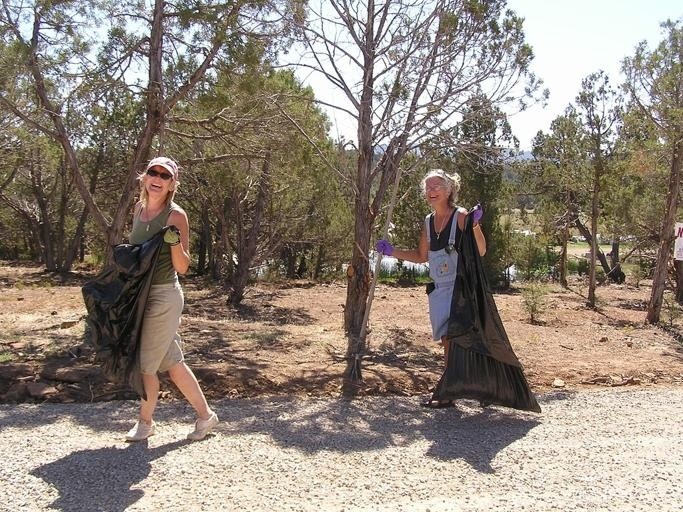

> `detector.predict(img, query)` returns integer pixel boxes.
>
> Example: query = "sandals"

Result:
[420,398,493,409]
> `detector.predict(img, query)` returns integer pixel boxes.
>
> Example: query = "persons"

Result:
[373,169,492,408]
[126,157,220,442]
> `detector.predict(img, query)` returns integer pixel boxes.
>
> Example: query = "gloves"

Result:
[471,203,484,223]
[375,239,394,257]
[163,224,182,246]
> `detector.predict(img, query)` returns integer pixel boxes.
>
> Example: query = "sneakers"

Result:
[124,420,157,440]
[187,413,219,440]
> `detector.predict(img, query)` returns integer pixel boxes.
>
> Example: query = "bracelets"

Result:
[472,222,479,230]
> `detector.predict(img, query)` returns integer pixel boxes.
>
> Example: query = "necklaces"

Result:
[434,205,450,240]
[146,200,167,232]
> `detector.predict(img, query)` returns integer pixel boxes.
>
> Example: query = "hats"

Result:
[146,156,179,176]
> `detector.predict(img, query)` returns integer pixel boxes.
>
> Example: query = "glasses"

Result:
[147,169,173,180]
[424,184,449,191]
